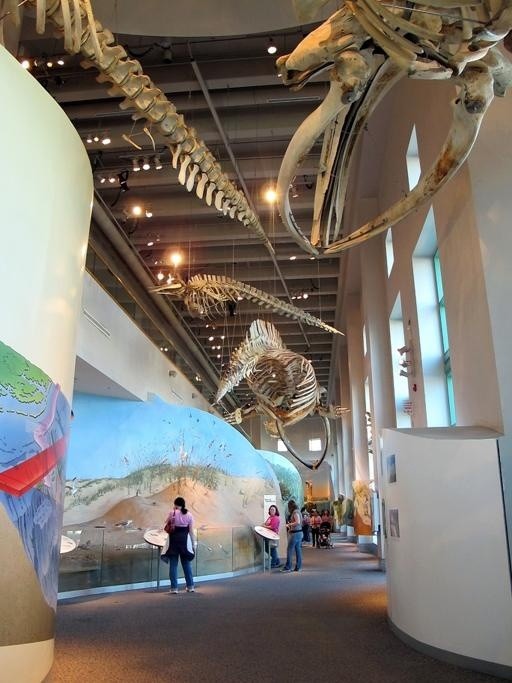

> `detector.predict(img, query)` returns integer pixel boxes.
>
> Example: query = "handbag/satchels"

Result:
[164,518,175,532]
[269,540,279,548]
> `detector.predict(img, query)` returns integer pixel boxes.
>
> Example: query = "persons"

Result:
[262,504,284,568]
[278,499,305,572]
[164,497,197,594]
[300,504,333,550]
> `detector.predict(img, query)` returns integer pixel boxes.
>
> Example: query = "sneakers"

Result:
[185,586,194,593]
[268,562,301,572]
[170,588,178,594]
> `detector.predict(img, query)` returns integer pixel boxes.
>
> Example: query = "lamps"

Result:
[81,134,189,291]
[398,338,416,379]
[204,322,225,368]
[290,287,310,300]
[20,53,67,72]
[262,180,299,203]
[266,36,278,54]
